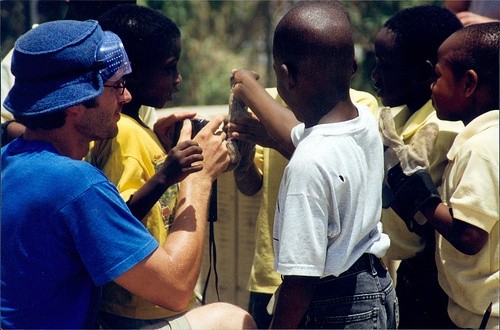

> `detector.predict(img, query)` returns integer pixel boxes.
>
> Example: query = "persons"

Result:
[0,0,500,330]
[0,19,259,330]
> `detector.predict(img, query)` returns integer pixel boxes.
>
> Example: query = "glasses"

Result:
[104,81,126,96]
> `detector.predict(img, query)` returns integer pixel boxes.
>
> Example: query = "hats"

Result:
[2,19,131,116]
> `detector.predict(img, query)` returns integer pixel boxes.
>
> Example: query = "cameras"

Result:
[172,119,209,146]
